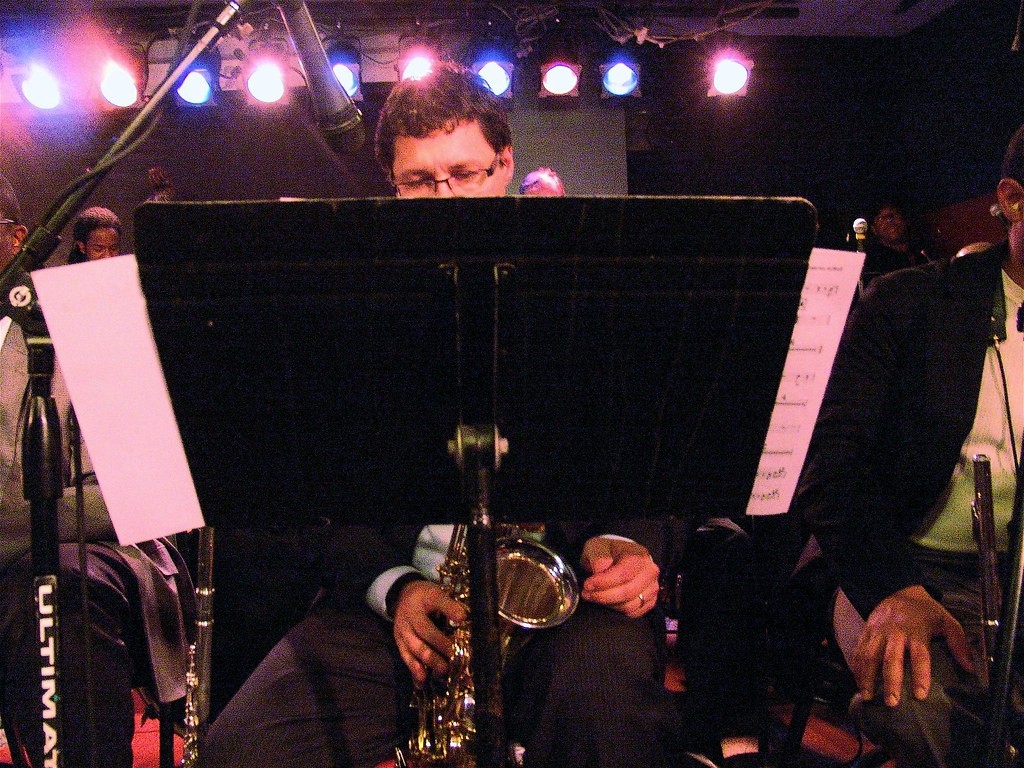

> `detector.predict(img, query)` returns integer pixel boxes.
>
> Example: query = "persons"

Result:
[866,201,928,281]
[194,62,678,768]
[808,120,1024,767]
[68,206,123,266]
[0,170,197,768]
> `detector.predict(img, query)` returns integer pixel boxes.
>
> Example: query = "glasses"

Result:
[386,151,499,200]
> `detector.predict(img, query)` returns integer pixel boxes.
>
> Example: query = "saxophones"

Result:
[392,519,579,768]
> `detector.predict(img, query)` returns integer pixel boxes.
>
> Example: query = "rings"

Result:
[639,594,645,605]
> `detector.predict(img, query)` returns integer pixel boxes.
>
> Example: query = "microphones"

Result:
[853,218,869,252]
[274,0,366,152]
[991,203,1011,233]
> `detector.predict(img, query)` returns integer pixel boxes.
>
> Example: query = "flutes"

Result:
[972,452,1001,689]
[182,527,215,768]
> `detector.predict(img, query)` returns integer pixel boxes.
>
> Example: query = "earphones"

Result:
[1013,202,1022,213]
